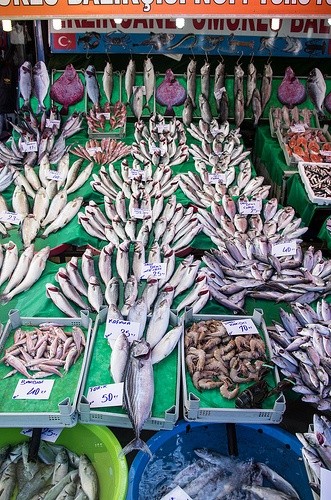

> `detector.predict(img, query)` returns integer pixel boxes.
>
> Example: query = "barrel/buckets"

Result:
[126,419,317,500]
[0,418,129,499]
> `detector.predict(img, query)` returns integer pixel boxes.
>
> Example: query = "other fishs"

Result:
[0,57,330,500]
